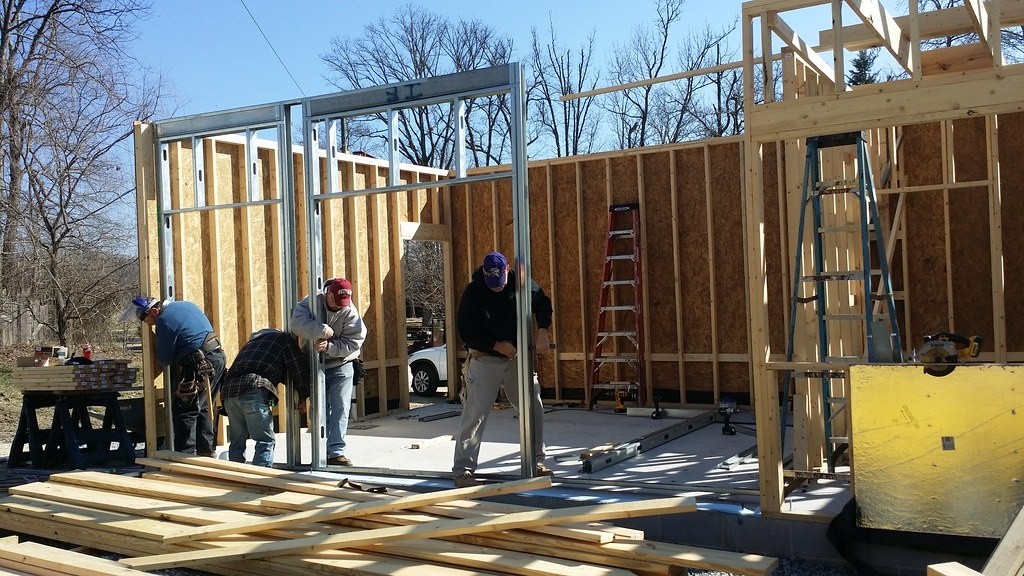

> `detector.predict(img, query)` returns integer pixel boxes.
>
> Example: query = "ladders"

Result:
[780,130,904,499]
[587,203,646,411]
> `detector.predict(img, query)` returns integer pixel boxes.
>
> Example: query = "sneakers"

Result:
[454,470,479,488]
[534,463,553,475]
[327,455,352,466]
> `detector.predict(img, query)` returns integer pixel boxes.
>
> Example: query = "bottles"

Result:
[58,346,66,366]
[82,341,92,359]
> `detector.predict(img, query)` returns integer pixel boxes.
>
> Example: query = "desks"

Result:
[7,390,135,470]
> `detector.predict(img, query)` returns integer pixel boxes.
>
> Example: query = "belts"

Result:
[207,347,222,355]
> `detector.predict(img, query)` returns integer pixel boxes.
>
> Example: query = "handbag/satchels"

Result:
[353,358,368,386]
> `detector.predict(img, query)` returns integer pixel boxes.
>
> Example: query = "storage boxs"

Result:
[34,345,67,367]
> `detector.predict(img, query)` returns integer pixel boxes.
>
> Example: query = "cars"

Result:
[408,342,449,397]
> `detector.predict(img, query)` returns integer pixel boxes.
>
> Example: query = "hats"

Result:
[482,252,508,288]
[330,277,353,306]
[134,296,159,321]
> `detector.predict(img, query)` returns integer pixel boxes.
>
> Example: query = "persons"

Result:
[451,252,554,488]
[132,298,226,458]
[221,329,310,469]
[290,278,368,466]
